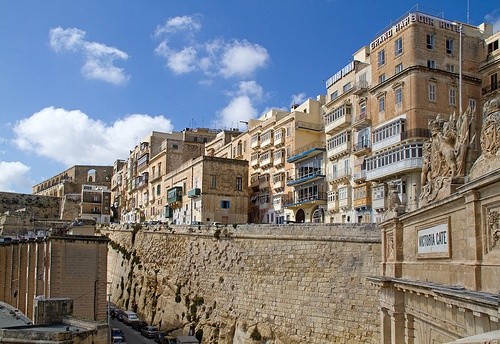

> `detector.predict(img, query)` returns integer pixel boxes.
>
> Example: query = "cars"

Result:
[125,221,225,227]
[163,335,175,344]
[141,326,160,339]
[109,304,145,344]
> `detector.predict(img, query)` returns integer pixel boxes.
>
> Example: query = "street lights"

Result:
[451,21,464,117]
